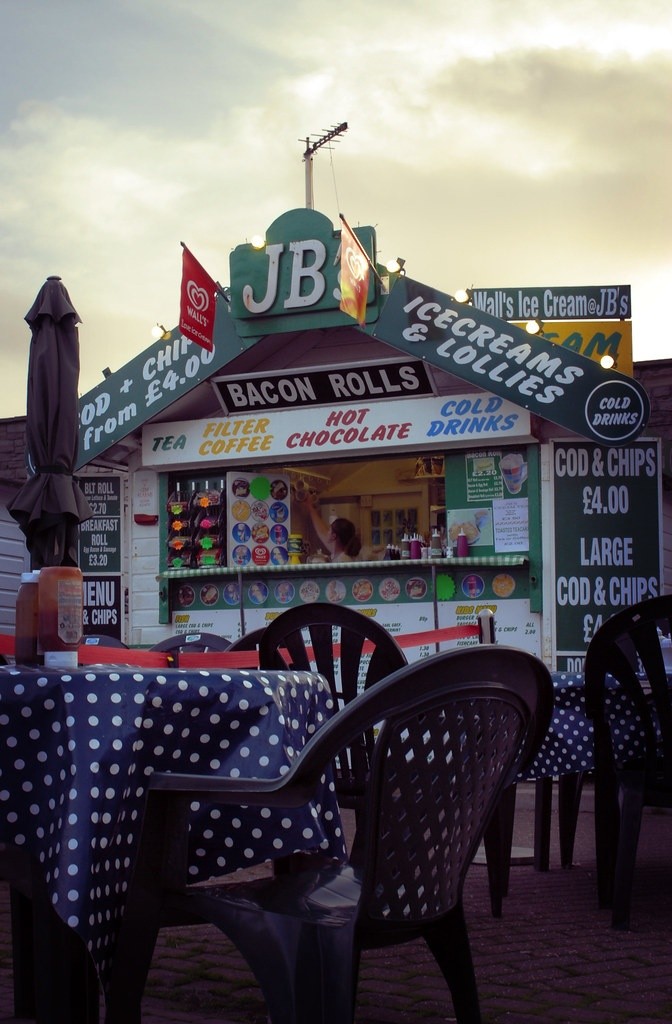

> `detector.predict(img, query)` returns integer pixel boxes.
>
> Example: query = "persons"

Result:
[304,496,361,563]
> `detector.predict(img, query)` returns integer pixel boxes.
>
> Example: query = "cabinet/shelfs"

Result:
[414,474,445,514]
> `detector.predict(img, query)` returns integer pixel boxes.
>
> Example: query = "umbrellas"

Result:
[6,277,94,567]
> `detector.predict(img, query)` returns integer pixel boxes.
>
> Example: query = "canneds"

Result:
[288,534,303,564]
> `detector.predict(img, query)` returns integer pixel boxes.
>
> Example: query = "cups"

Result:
[502,462,529,494]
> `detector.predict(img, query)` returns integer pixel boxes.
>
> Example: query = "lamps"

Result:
[387,257,406,276]
[526,317,545,336]
[454,287,474,305]
[600,352,619,369]
[151,322,167,340]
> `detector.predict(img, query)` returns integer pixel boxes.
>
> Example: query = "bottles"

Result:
[15,572,44,666]
[38,566,83,666]
[383,533,427,560]
[468,576,476,597]
[457,528,469,557]
[431,528,442,558]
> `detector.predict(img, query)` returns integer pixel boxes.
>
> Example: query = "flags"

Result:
[180,243,220,353]
[336,213,371,330]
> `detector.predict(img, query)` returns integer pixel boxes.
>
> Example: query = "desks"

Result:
[0,665,350,1024]
[528,671,672,871]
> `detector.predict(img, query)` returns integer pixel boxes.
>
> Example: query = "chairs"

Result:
[79,629,269,652]
[584,594,672,930]
[106,644,556,1024]
[259,602,515,918]
